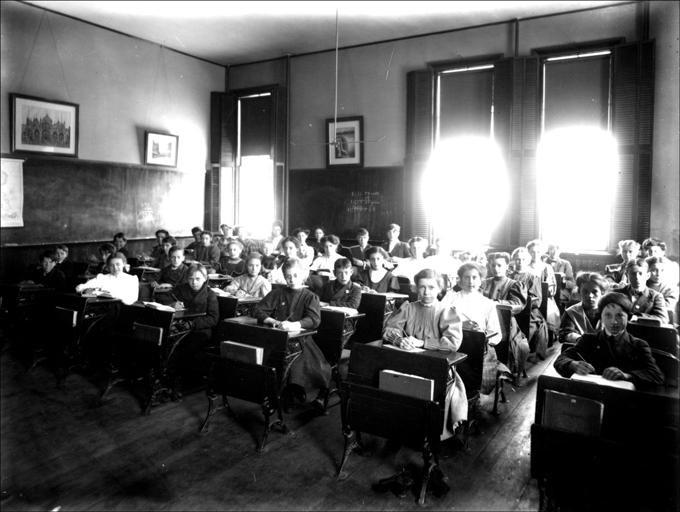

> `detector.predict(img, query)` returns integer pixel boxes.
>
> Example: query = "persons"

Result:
[380,239,575,396]
[556,237,680,389]
[24,223,463,334]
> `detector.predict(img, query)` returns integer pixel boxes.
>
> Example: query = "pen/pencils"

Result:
[402,330,410,340]
[576,351,596,374]
[357,280,365,286]
[170,292,179,301]
[463,313,471,321]
[234,282,241,290]
[284,312,294,321]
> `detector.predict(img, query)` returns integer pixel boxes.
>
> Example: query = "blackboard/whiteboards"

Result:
[288,165,406,243]
[0,152,205,248]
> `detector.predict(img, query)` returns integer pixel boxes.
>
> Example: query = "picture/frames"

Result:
[323,115,364,167]
[144,129,177,170]
[7,90,79,156]
